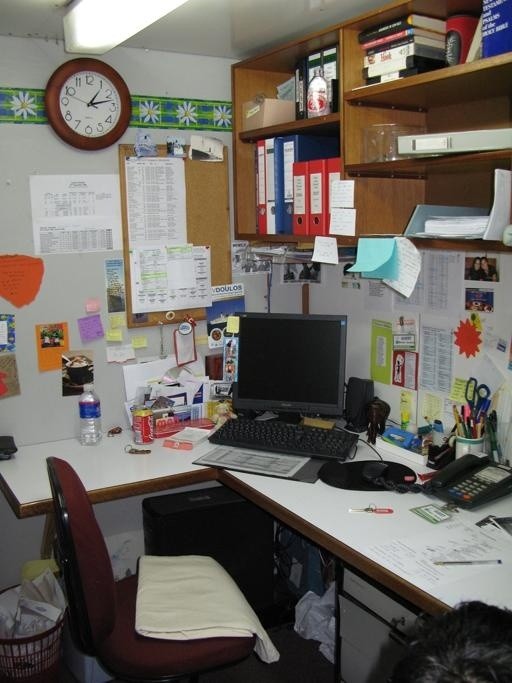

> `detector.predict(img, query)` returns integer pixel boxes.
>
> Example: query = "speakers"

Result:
[232,381,257,418]
[344,377,374,434]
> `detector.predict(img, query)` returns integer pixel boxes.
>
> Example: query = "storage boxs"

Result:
[238,94,294,134]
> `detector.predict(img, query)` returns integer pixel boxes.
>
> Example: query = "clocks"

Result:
[43,56,133,151]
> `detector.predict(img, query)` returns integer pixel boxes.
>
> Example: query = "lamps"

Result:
[61,0,187,54]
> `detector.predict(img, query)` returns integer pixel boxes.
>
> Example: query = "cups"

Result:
[455,434,485,461]
[64,359,93,384]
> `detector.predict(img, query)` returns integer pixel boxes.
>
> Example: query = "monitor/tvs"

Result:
[237,311,347,425]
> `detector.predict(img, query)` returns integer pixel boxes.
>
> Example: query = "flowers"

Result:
[174,101,198,127]
[9,91,38,119]
[137,100,161,124]
[213,104,232,128]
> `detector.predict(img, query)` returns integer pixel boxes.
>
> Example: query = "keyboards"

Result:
[208,418,360,461]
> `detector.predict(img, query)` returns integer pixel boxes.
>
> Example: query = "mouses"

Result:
[361,462,388,481]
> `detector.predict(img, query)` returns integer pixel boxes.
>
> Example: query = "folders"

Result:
[258,135,341,236]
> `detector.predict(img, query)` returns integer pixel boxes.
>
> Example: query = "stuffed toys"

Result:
[207,402,238,437]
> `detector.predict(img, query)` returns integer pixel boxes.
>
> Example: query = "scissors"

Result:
[465,377,490,418]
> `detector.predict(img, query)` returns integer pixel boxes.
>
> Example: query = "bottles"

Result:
[78,383,101,444]
[306,68,330,119]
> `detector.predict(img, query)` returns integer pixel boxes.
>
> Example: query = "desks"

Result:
[0,406,512,682]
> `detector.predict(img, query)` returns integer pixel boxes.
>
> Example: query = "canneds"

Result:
[133,406,153,445]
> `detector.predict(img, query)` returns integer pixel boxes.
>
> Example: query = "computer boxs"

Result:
[142,486,274,631]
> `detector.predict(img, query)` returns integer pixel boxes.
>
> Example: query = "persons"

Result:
[468,257,483,280]
[225,339,234,358]
[225,360,233,383]
[480,257,497,282]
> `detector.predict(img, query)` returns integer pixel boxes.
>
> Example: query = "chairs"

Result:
[41,454,259,682]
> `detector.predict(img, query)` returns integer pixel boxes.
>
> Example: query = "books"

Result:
[350,0,512,92]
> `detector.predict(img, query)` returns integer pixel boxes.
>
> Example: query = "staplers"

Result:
[427,445,455,470]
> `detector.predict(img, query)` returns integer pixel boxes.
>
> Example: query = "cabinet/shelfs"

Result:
[345,0,512,254]
[227,25,351,249]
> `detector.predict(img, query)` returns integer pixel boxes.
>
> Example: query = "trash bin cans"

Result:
[0,584,65,683]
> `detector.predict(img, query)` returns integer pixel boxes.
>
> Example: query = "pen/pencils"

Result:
[453,405,500,464]
[434,561,502,565]
